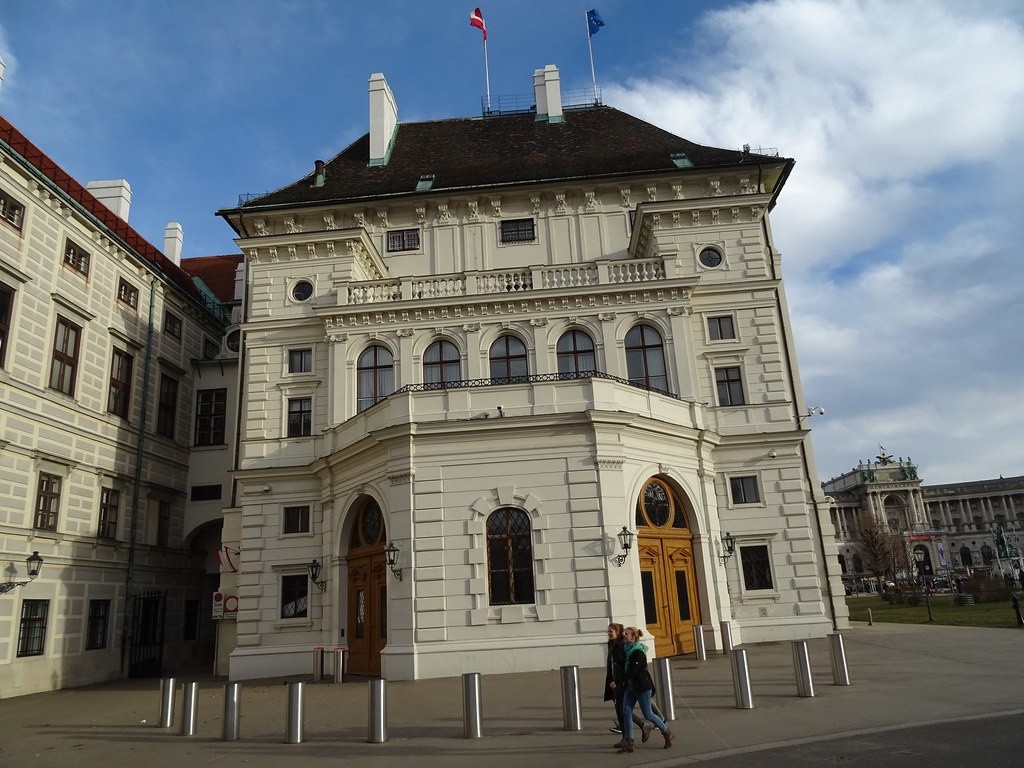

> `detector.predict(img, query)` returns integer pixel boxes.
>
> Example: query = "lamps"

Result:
[0,551,44,594]
[384,542,402,582]
[308,558,327,593]
[719,532,737,566]
[618,525,634,567]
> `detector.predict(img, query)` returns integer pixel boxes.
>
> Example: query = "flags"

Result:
[469,7,487,42]
[586,9,606,39]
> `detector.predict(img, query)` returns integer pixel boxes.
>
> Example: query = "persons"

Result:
[603,624,667,749]
[616,627,674,754]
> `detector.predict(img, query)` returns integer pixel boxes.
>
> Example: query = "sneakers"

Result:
[609,725,622,734]
[653,718,668,730]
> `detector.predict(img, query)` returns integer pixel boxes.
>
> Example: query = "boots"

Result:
[617,742,634,754]
[661,728,676,749]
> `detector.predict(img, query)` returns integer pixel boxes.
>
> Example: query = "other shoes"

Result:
[641,723,652,743]
[614,741,634,748]
